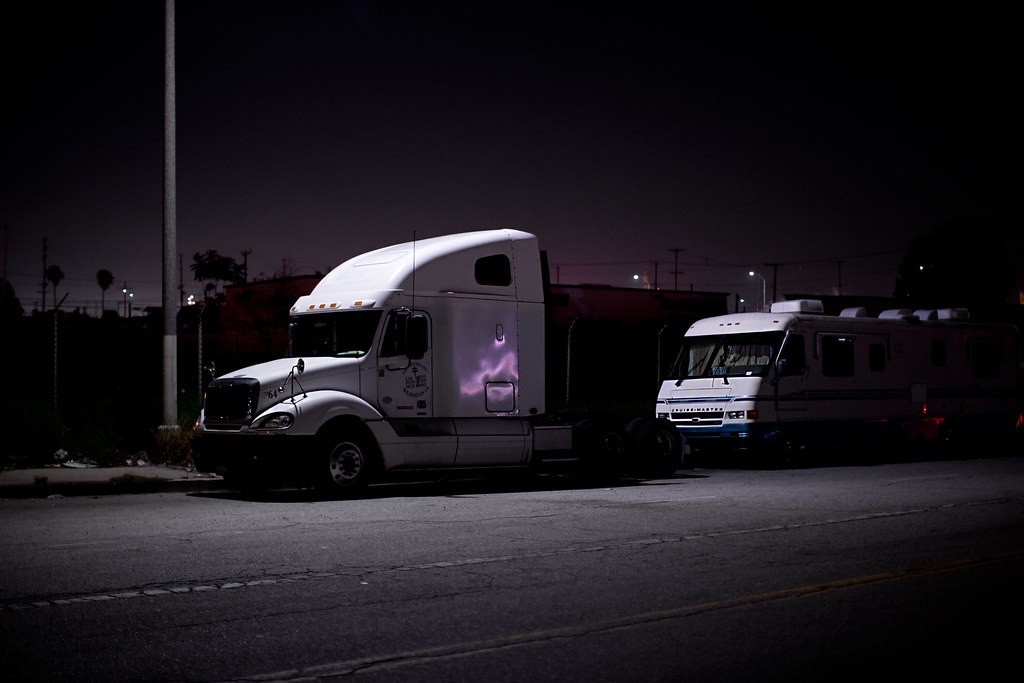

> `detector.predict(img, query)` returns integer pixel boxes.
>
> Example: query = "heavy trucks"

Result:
[188,228,683,500]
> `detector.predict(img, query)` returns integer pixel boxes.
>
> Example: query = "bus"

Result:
[653,299,1021,464]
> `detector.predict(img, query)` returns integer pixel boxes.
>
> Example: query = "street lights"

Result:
[129,293,134,317]
[748,270,766,309]
[122,288,127,318]
